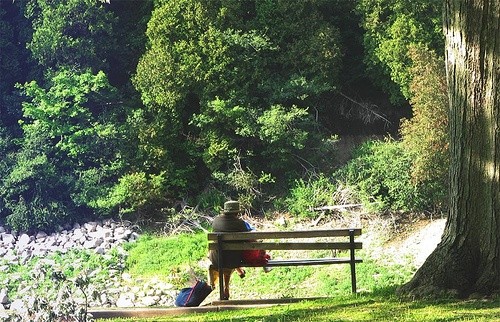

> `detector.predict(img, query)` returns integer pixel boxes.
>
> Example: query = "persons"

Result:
[207,201,249,300]
[243,222,274,273]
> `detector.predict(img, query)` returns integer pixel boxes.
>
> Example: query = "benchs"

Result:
[207,229,363,305]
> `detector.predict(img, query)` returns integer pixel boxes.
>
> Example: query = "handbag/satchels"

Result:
[174,281,212,307]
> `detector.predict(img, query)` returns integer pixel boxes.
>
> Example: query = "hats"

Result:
[223,201,242,214]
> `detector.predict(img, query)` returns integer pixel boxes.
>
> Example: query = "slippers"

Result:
[239,268,246,278]
[224,288,230,300]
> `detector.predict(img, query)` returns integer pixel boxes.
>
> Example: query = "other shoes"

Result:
[264,267,274,273]
